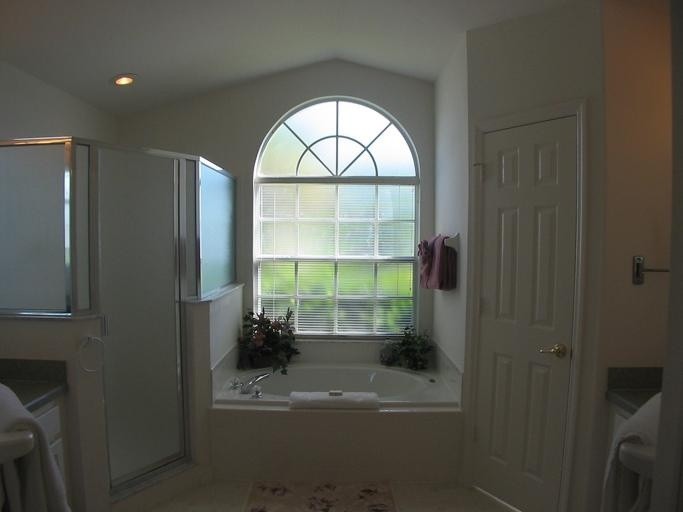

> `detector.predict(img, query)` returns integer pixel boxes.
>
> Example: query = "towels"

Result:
[0,383,73,512]
[288,388,380,410]
[416,234,457,292]
[594,391,663,511]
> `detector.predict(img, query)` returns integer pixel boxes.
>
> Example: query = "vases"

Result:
[397,350,408,365]
[248,349,270,369]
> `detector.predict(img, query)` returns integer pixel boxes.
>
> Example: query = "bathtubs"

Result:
[212,362,457,407]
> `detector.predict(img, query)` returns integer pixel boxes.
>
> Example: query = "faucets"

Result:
[231,372,272,400]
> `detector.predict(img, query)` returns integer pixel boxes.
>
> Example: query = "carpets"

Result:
[243,479,399,512]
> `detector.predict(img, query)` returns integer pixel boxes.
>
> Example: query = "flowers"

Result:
[234,303,301,375]
[378,324,437,373]
